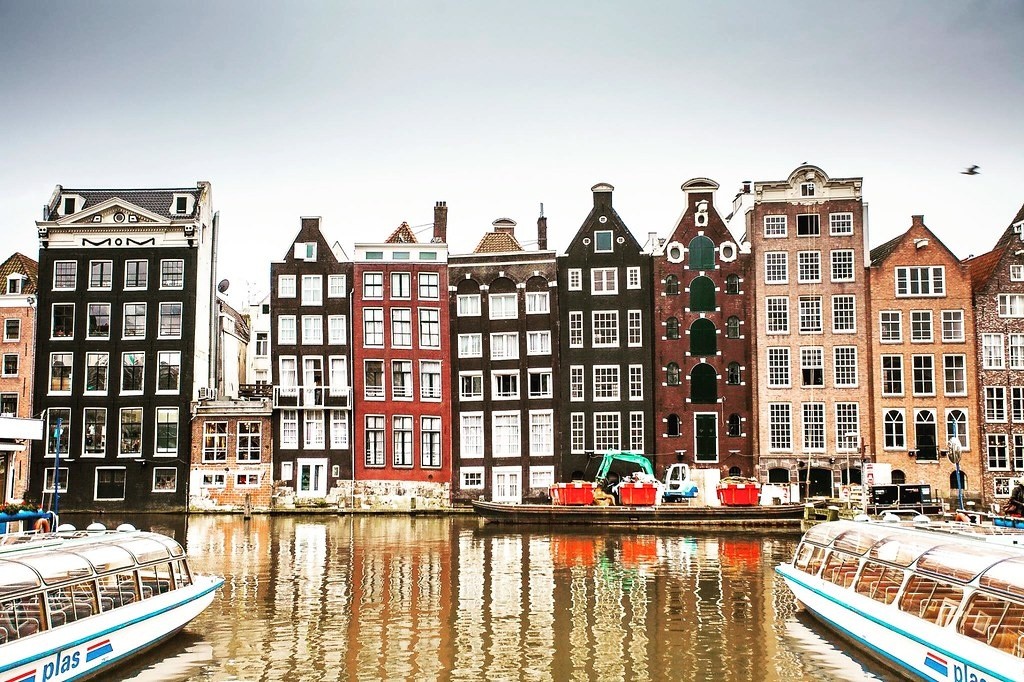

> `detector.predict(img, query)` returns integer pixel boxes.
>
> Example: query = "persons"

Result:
[1003,476,1024,518]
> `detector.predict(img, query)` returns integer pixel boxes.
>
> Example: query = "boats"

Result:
[775,518,1024,682]
[0,523,224,682]
[470,495,834,525]
[828,480,951,520]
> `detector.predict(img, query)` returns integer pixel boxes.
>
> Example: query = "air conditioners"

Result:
[200,387,213,399]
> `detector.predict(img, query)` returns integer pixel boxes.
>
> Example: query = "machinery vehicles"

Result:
[592,448,700,507]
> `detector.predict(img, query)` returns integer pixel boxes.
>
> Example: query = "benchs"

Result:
[795,548,1024,658]
[0,581,170,645]
